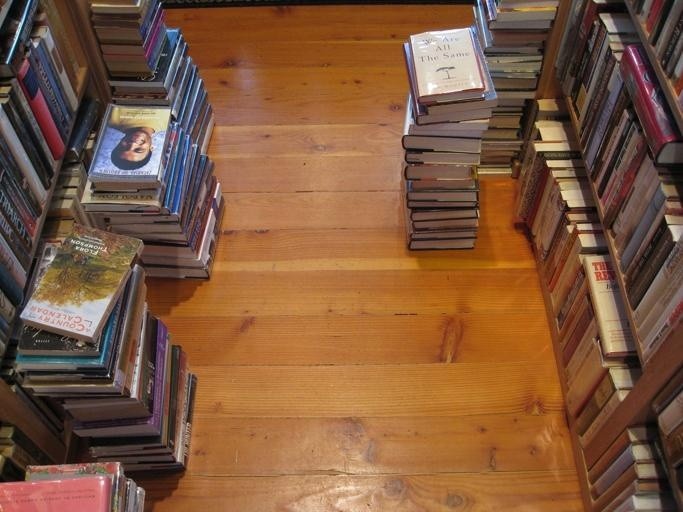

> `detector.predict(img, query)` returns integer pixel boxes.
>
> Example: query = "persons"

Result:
[110,105,170,170]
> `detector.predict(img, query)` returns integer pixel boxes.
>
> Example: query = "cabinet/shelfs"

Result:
[512,0,682,510]
[1,1,113,465]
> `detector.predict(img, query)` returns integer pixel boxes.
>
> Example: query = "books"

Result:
[86,0,215,155]
[549,0,682,373]
[14,223,199,479]
[0,458,145,512]
[472,0,559,169]
[398,25,498,251]
[79,103,225,279]
[512,96,641,449]
[585,371,682,512]
[0,372,71,484]
[1,0,98,372]
